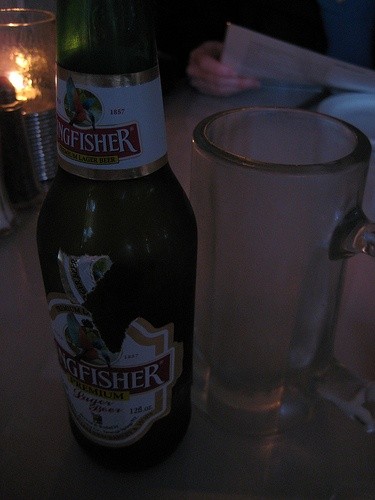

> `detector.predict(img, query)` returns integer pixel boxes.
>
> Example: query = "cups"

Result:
[192,107,375,439]
[0,177,18,238]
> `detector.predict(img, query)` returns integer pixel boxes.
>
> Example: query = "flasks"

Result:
[0,75,41,211]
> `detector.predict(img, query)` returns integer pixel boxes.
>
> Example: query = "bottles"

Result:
[38,0,197,470]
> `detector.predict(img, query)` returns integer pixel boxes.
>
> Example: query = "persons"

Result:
[90,0,331,97]
